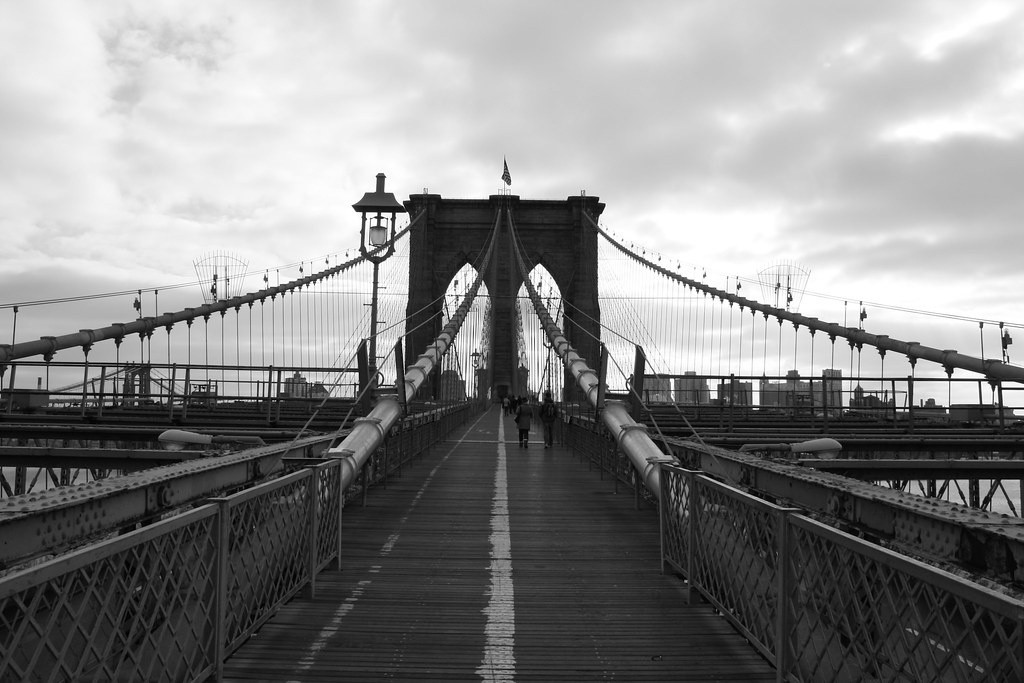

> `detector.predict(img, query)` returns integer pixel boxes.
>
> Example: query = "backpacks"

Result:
[544,403,554,417]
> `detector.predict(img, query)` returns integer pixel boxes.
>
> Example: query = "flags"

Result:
[501,160,511,186]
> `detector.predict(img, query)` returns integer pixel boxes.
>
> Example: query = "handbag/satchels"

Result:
[514,406,521,424]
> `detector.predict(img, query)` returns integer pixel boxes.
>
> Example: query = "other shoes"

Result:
[519,443,523,446]
[524,440,527,447]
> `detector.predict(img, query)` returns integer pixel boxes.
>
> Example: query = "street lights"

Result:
[351,173,407,388]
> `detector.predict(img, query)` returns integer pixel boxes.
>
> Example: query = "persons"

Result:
[503,395,522,416]
[515,397,533,448]
[539,393,557,448]
